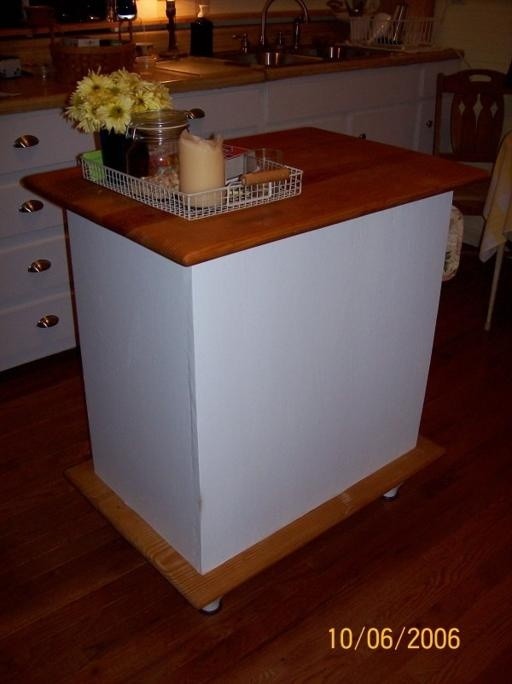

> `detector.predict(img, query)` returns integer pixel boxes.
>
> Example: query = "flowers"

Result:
[63,66,172,135]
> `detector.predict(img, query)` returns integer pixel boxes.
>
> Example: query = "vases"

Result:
[100,122,150,185]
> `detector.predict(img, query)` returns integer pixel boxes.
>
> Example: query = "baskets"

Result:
[77,144,303,223]
[50,18,137,83]
[350,15,443,54]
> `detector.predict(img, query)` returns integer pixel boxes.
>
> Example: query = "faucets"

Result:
[260,0,308,45]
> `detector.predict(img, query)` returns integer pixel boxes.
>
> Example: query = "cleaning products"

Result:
[190,4,214,57]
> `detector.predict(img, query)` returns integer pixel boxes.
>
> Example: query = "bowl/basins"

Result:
[48,45,138,89]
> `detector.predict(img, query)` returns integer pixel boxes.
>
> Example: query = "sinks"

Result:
[286,43,399,63]
[215,50,320,72]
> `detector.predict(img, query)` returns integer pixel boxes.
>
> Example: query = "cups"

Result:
[0,55,22,80]
[242,148,283,196]
[137,42,153,55]
[134,57,159,79]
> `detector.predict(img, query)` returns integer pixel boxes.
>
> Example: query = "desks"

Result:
[19,126,489,615]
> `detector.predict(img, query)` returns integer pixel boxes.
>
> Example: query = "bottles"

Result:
[190,16,215,58]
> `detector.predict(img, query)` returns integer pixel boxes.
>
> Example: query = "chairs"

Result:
[432,68,511,215]
[460,131,512,332]
